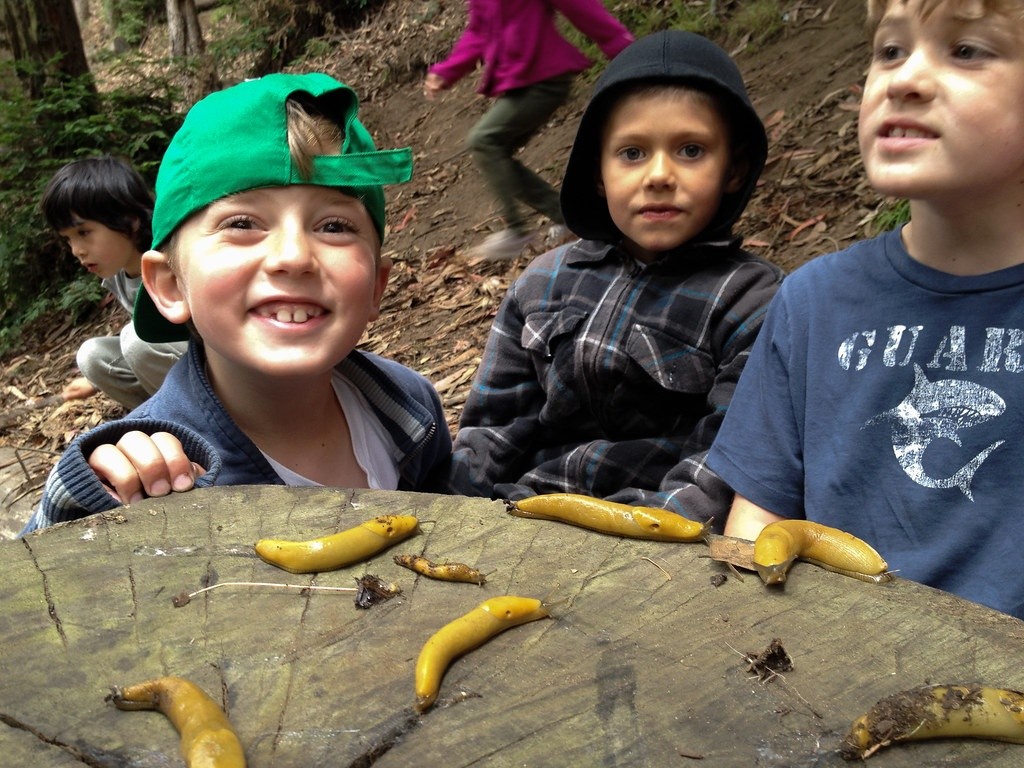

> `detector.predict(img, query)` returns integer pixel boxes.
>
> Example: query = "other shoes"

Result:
[484,227,537,260]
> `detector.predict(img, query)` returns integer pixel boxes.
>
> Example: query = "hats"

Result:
[133,72,415,344]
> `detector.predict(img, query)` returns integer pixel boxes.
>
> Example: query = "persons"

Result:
[423,1,635,259]
[704,0,1024,621]
[41,158,190,416]
[19,73,453,537]
[453,30,785,540]
